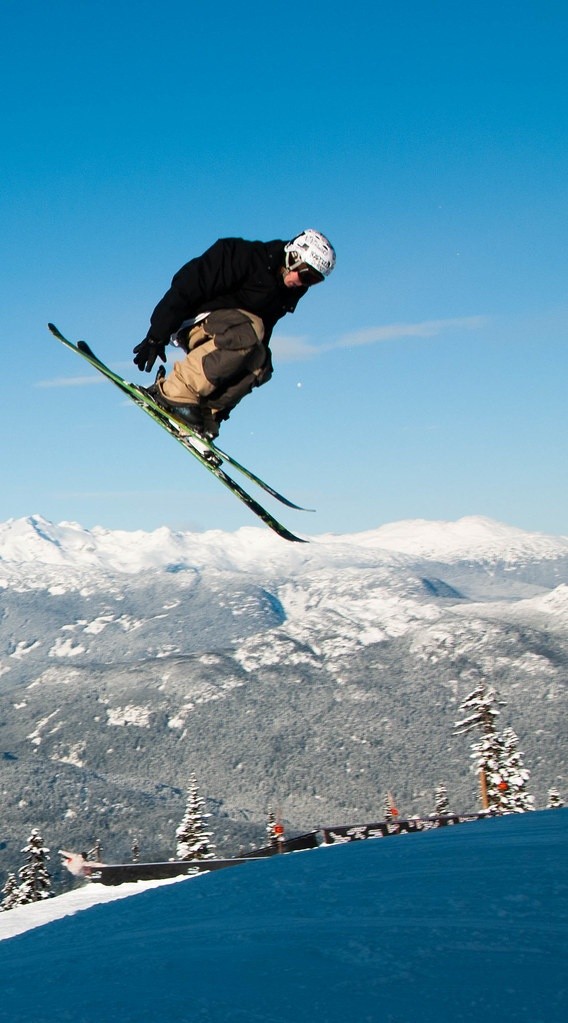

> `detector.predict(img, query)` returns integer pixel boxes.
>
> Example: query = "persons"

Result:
[131,228,337,437]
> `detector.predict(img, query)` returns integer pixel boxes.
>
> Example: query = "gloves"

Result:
[133,333,166,373]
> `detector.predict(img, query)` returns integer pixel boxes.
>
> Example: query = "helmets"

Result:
[284,229,336,279]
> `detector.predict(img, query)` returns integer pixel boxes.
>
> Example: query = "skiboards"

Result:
[46,321,318,544]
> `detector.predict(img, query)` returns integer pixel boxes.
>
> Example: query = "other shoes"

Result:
[147,383,204,432]
[200,407,219,439]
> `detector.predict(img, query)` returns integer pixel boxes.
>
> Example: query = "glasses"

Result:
[298,269,323,288]
